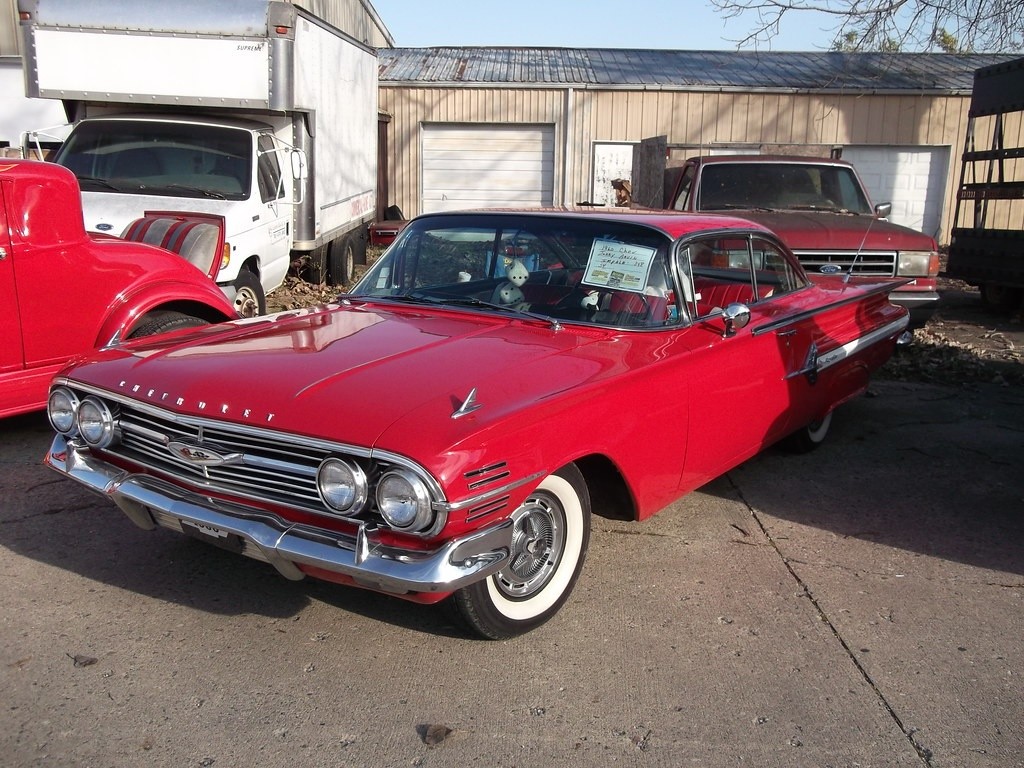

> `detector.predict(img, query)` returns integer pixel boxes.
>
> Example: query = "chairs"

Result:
[542,268,608,315]
[609,281,668,325]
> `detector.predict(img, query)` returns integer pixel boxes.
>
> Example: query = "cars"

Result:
[45,207,914,643]
[0,157,241,418]
[665,155,941,330]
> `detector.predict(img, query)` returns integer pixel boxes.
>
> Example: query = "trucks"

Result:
[16,0,380,320]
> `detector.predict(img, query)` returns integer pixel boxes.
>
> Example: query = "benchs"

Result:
[672,272,778,323]
